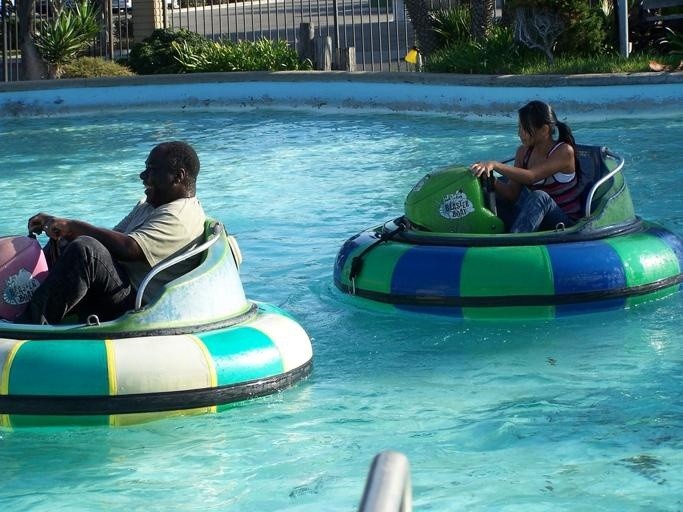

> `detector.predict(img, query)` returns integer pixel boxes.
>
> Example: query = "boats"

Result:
[331,143,683,326]
[1,219,317,428]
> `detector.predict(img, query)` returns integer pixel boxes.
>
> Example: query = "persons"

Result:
[469,98,583,235]
[0,139,206,325]
